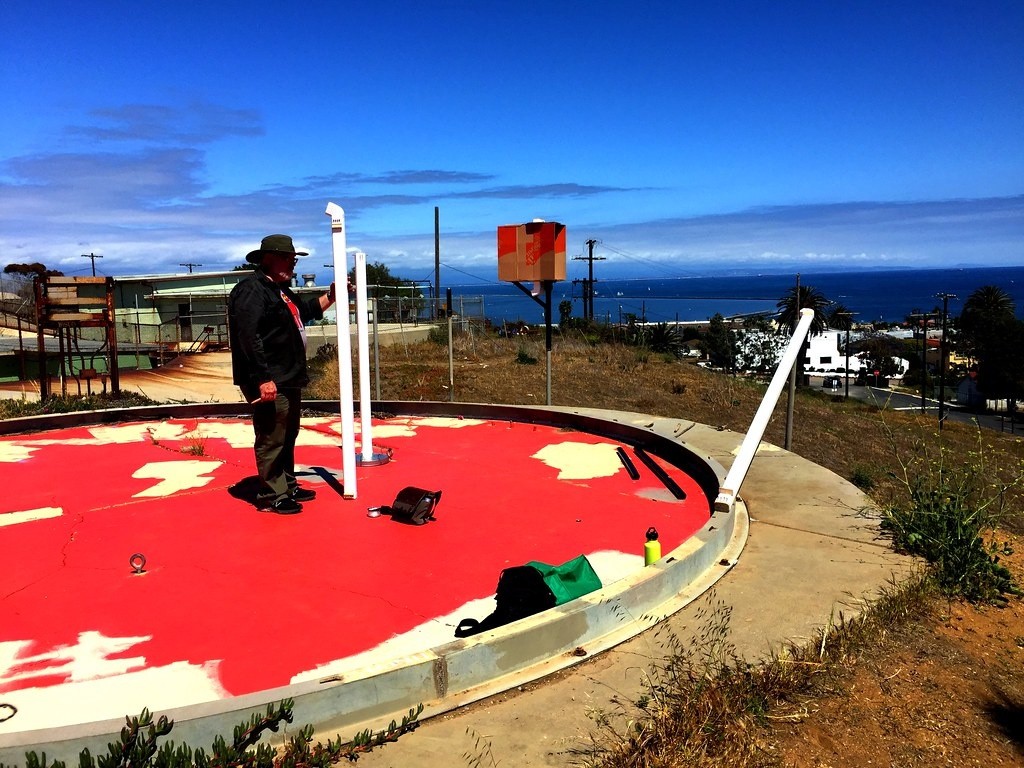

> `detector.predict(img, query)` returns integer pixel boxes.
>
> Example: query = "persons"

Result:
[228,234,355,514]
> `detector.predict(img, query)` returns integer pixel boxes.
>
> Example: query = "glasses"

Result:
[279,254,299,264]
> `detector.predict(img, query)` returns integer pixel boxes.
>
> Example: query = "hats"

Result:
[245,234,309,264]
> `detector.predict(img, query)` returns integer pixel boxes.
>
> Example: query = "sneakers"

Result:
[259,497,303,514]
[288,486,316,501]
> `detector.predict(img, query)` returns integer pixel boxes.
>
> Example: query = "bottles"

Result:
[644,527,662,567]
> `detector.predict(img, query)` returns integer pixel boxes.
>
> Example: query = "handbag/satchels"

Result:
[527,553,600,605]
[376,485,442,525]
[447,565,558,637]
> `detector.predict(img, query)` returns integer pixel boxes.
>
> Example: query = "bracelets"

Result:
[327,291,332,301]
